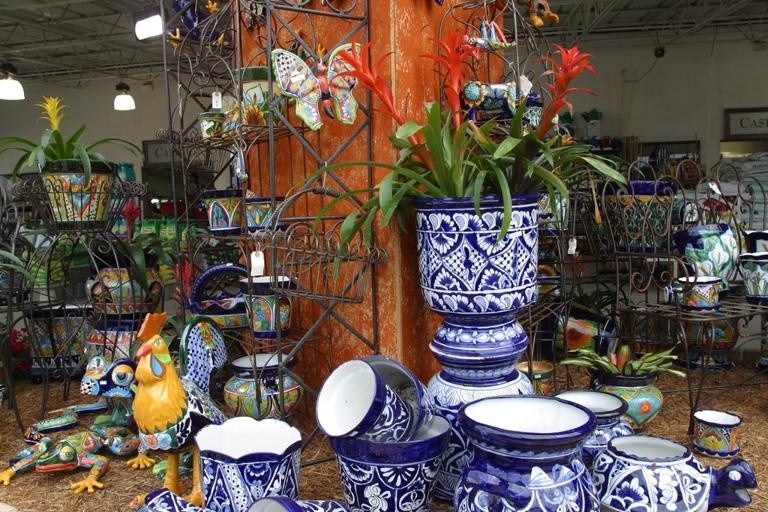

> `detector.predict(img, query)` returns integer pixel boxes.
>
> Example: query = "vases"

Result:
[0,1,767,509]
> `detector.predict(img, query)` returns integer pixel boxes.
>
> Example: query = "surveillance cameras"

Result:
[654,47,665,57]
